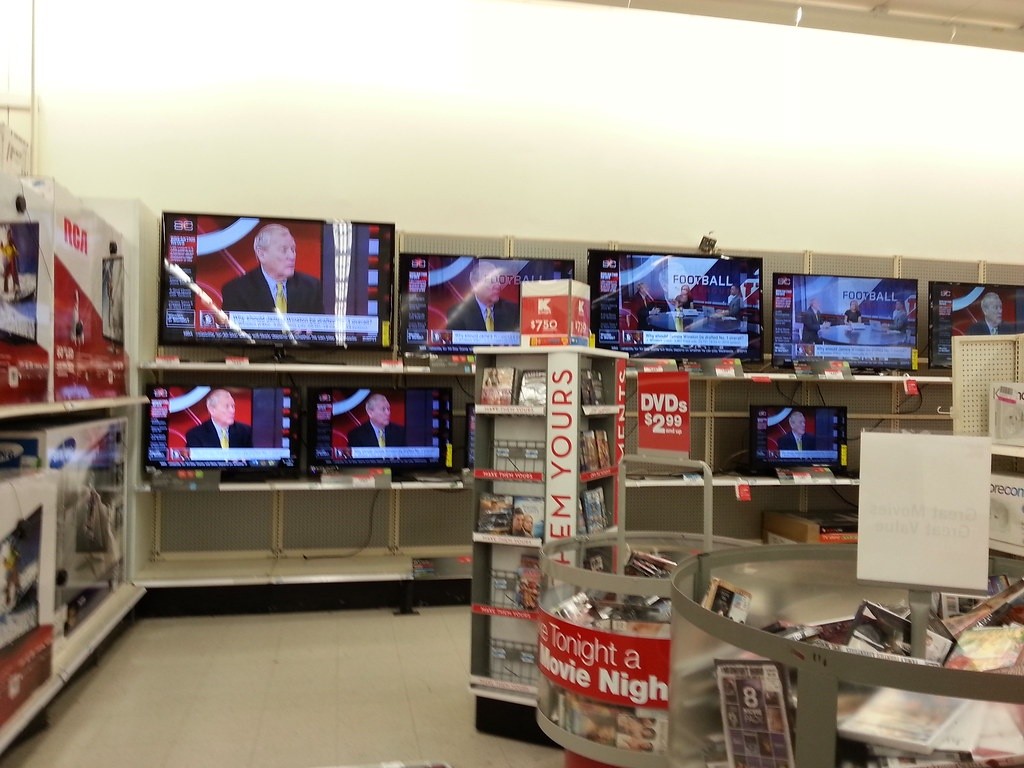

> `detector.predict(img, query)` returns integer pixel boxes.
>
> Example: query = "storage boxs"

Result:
[988,382,1024,447]
[579,430,612,473]
[0,175,130,726]
[477,491,545,538]
[482,365,547,406]
[580,486,609,534]
[989,471,1024,557]
[581,369,606,406]
[699,574,1024,768]
[762,508,858,546]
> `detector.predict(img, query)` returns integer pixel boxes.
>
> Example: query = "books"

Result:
[700,575,1024,768]
[551,547,677,752]
[480,367,611,611]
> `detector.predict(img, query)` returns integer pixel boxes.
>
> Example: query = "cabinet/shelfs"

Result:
[0,395,146,756]
[399,231,713,580]
[466,345,629,752]
[951,333,1024,559]
[124,362,430,616]
[708,245,953,487]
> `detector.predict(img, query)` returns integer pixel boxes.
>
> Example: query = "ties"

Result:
[275,282,287,312]
[485,308,494,331]
[221,428,229,449]
[798,437,802,450]
[379,429,385,447]
[993,328,998,335]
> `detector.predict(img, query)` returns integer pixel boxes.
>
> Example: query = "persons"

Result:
[802,297,907,345]
[186,388,253,448]
[522,513,533,534]
[347,394,405,447]
[778,412,816,450]
[966,292,1013,335]
[447,262,520,332]
[222,224,323,314]
[632,283,746,331]
[511,508,523,537]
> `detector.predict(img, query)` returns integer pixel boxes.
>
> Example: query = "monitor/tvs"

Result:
[0,505,42,656]
[0,219,40,346]
[100,254,126,356]
[75,429,116,577]
[148,212,1024,486]
